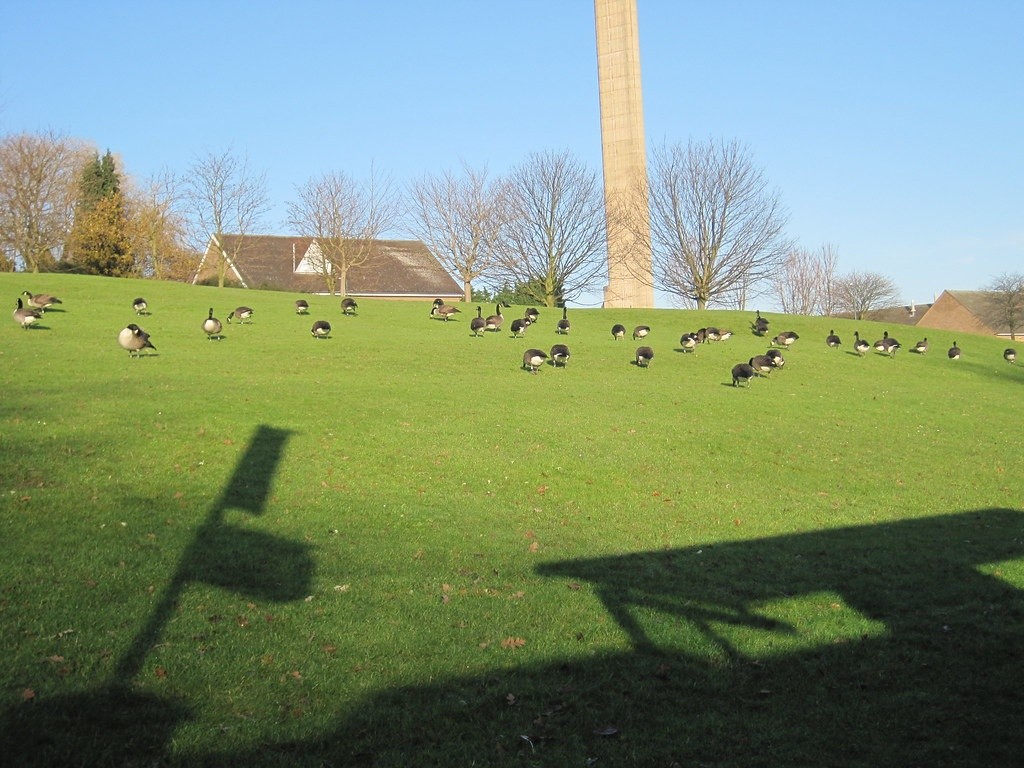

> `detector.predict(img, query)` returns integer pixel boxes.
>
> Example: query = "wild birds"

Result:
[824,329,961,362]
[429,296,571,375]
[117,298,157,357]
[201,305,254,342]
[611,323,735,368]
[1003,346,1018,365]
[295,298,360,339]
[730,308,799,388]
[12,291,63,331]
